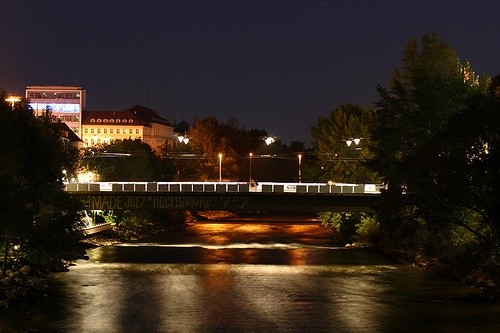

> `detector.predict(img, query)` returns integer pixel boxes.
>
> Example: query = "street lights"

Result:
[249,153,252,182]
[218,153,222,182]
[297,155,302,182]
